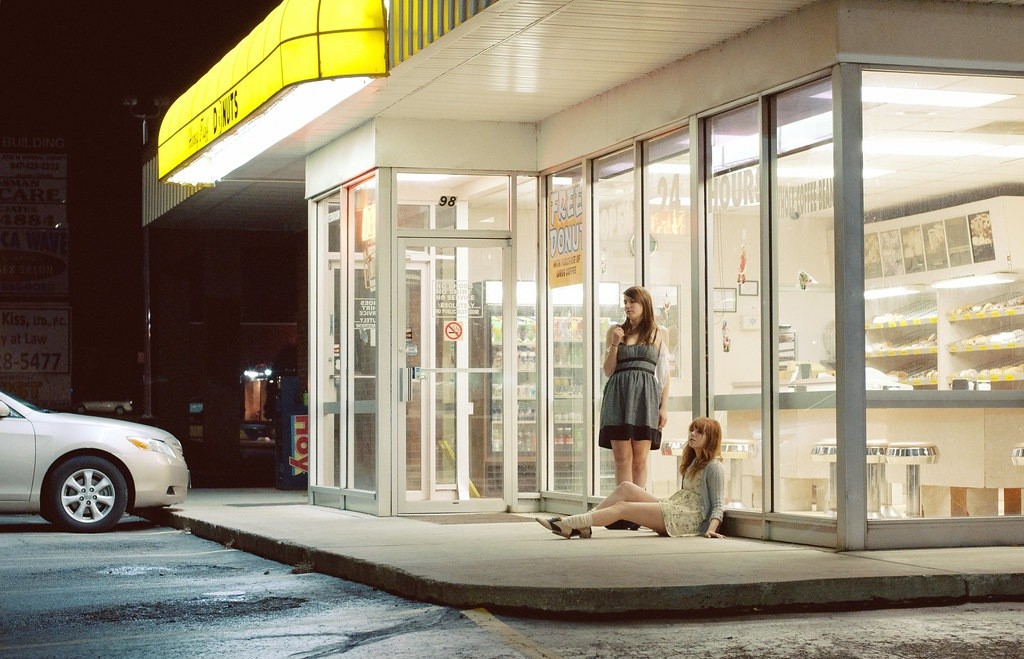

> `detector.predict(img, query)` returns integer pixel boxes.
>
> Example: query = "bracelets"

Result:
[610,344,617,351]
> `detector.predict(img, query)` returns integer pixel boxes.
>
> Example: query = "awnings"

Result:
[159,0,389,185]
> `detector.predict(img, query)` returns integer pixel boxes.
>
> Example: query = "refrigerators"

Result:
[470,281,621,497]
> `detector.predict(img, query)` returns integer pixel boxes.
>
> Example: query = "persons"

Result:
[536,417,726,540]
[599,286,670,530]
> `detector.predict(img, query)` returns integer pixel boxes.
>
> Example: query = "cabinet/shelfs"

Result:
[865,304,1024,389]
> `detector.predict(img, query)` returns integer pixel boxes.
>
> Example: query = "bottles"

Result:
[491,316,584,457]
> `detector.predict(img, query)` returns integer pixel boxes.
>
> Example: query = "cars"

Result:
[0,390,193,531]
[78,394,134,416]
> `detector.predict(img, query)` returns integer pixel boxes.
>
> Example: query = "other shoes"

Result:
[535,516,572,536]
[606,518,640,531]
[553,527,592,538]
